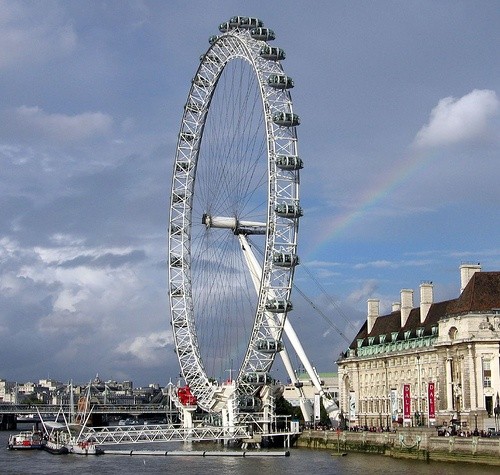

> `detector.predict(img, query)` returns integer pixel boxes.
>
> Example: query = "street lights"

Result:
[474,412,479,436]
[342,411,390,433]
[451,413,455,436]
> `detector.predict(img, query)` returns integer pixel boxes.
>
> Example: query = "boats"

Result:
[9,429,45,451]
